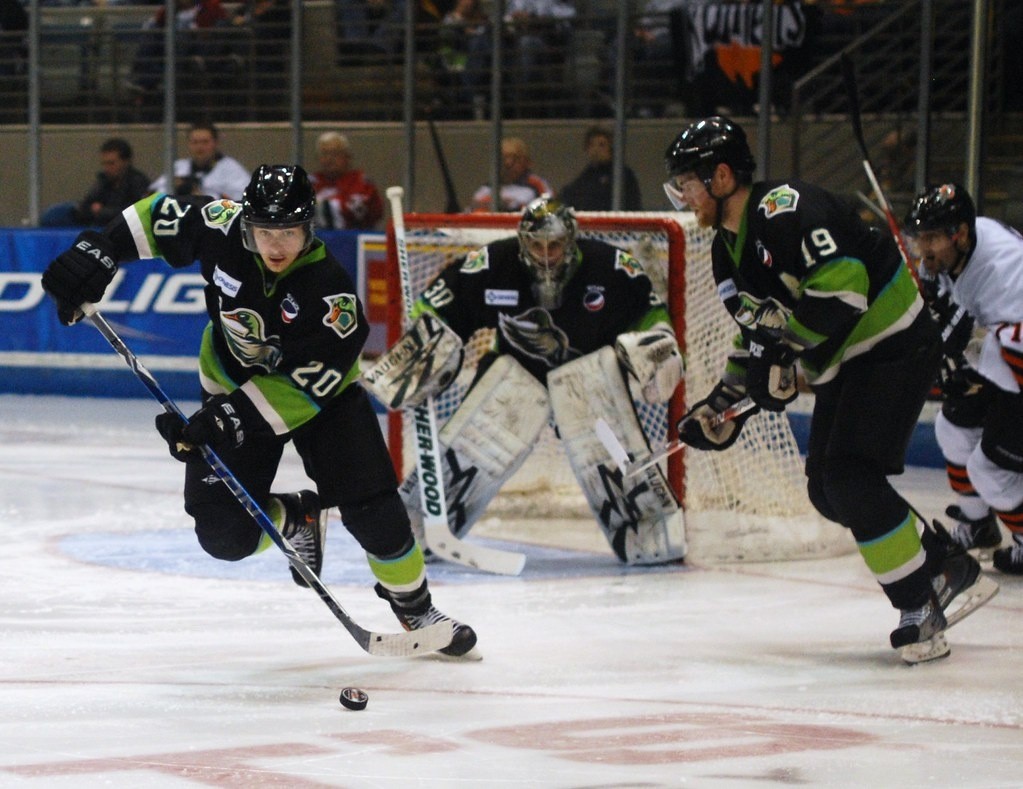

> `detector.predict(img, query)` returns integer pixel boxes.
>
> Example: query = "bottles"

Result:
[474,90,489,120]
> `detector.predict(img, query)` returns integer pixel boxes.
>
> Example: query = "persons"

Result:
[558,125,643,212]
[304,131,382,232]
[462,135,556,212]
[42,165,476,658]
[908,183,1022,578]
[0,0,889,121]
[75,136,156,229]
[359,193,686,565]
[666,117,983,648]
[149,122,252,201]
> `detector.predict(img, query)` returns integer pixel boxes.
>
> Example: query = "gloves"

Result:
[675,381,760,450]
[745,336,798,413]
[154,392,246,464]
[926,284,975,358]
[42,229,119,327]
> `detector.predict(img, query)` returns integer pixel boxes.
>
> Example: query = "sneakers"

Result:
[945,505,1002,561]
[889,586,950,666]
[992,536,1023,578]
[270,489,328,587]
[928,518,999,626]
[374,576,484,661]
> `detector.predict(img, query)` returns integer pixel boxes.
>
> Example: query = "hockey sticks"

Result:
[594,375,788,477]
[862,158,946,384]
[386,185,526,576]
[79,301,454,657]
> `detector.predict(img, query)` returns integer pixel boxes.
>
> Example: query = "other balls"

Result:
[340,688,369,711]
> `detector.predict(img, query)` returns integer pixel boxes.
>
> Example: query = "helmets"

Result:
[903,180,976,242]
[516,196,578,285]
[241,163,315,228]
[665,114,755,189]
